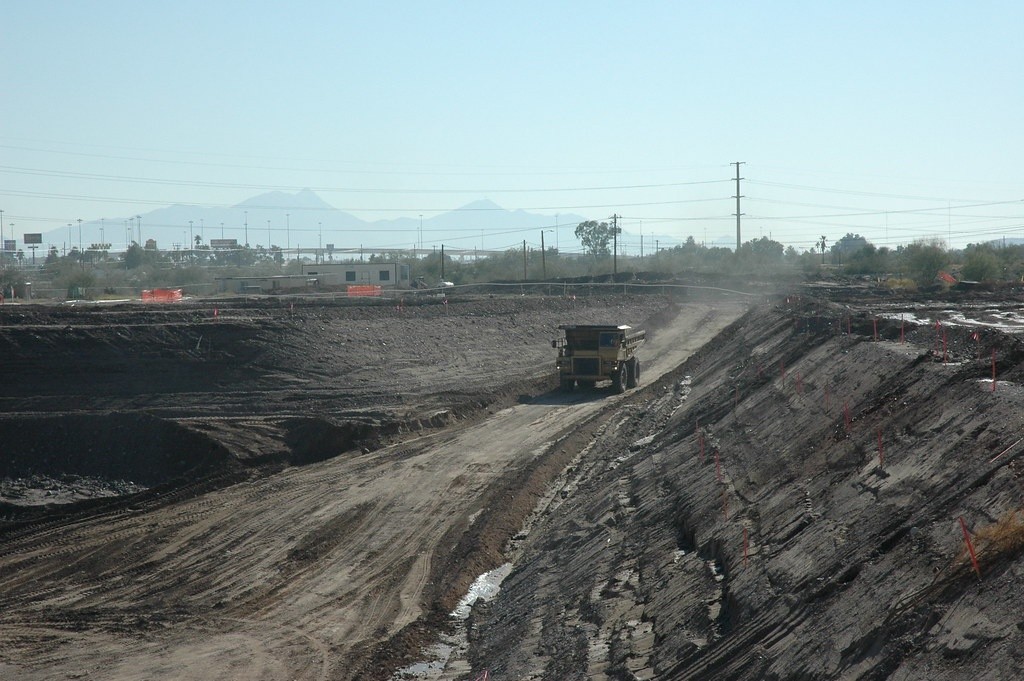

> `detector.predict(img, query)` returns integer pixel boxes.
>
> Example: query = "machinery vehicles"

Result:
[551,324,647,392]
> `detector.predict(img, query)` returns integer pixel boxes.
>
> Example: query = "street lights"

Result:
[0,209,423,263]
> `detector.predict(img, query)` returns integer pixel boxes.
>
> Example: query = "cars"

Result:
[436,282,453,287]
